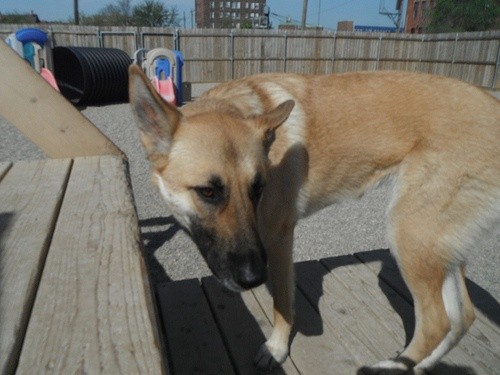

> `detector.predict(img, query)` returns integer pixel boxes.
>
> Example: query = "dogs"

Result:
[127,62,499,375]
